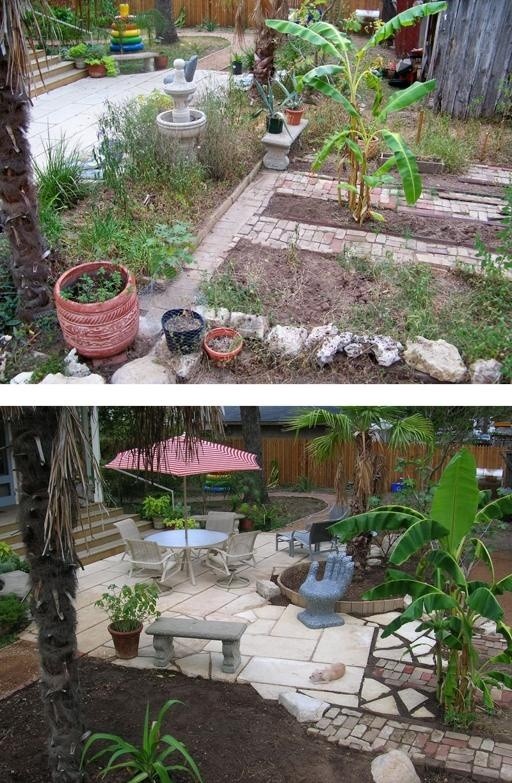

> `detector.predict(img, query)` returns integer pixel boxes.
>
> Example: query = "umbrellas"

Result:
[101,430,263,547]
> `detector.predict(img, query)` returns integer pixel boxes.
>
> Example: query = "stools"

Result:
[276,530,306,556]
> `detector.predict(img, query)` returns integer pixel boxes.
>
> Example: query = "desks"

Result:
[144,530,228,585]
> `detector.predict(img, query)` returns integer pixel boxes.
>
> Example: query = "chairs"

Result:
[124,538,181,583]
[113,518,142,560]
[207,530,261,589]
[187,511,236,561]
[291,520,339,560]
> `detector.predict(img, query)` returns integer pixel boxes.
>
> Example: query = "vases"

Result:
[241,518,255,530]
[355,135,379,159]
[202,328,243,368]
[154,55,168,68]
[161,309,203,354]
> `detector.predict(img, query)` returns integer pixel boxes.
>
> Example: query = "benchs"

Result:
[146,618,248,673]
[261,119,308,171]
[192,514,244,534]
[111,52,158,73]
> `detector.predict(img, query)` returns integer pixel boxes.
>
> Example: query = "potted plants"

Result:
[253,78,298,133]
[231,51,243,75]
[53,262,139,358]
[94,583,162,659]
[257,504,279,531]
[143,495,170,529]
[85,58,116,78]
[273,69,304,125]
[69,42,88,68]
